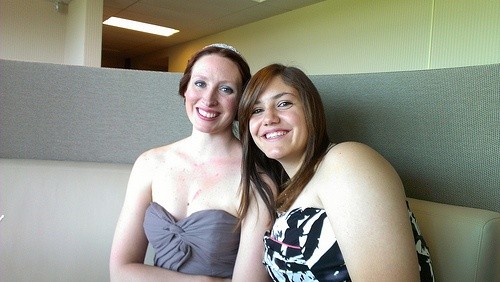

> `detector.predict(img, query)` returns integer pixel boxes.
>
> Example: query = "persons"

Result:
[110,43,278,282]
[232,63,435,282]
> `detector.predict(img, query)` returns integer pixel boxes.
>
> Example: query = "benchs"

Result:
[0,161,500,281]
